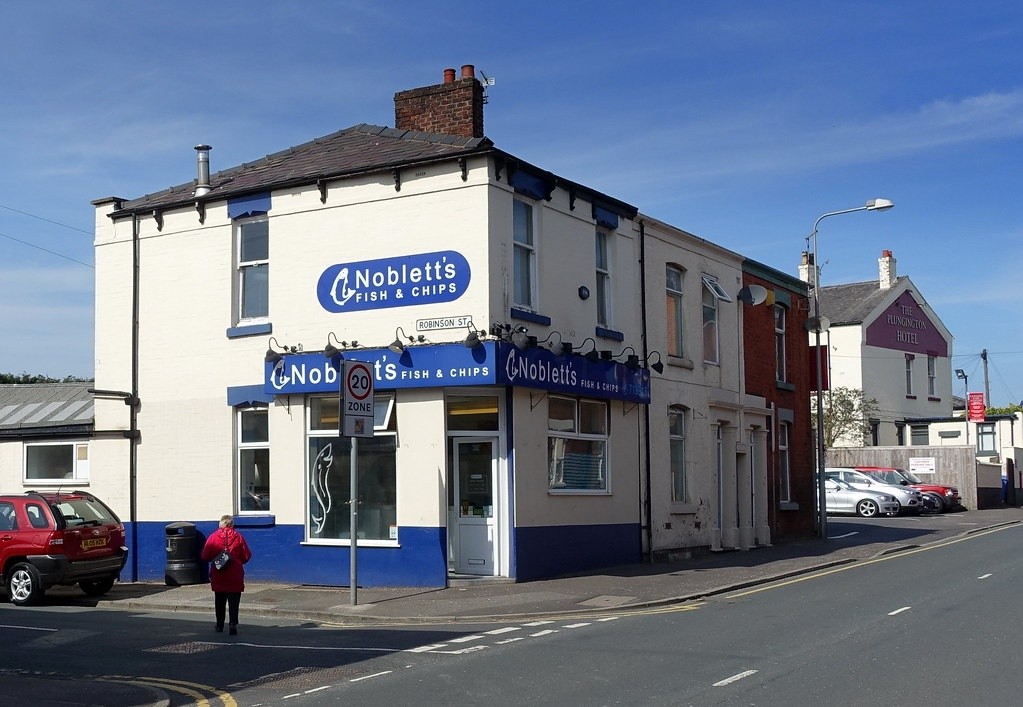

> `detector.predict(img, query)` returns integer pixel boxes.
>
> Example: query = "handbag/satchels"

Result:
[211,531,232,572]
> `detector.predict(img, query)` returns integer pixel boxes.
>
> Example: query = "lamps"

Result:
[528,330,565,356]
[465,321,487,348]
[601,346,641,369]
[562,338,600,363]
[324,332,349,359]
[628,350,664,374]
[502,323,530,350]
[265,337,291,362]
[388,327,418,354]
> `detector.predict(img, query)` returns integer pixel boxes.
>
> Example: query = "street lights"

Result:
[813,199,895,540]
[953,368,969,445]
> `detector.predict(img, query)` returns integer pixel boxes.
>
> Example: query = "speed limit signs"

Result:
[343,360,376,416]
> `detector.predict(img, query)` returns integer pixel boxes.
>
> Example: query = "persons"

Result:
[201,515,252,636]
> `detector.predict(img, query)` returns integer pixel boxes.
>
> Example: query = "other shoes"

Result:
[229,626,237,635]
[215,625,223,632]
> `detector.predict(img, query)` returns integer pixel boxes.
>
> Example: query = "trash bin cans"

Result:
[165,522,199,585]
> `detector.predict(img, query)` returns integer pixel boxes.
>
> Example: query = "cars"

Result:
[813,467,961,519]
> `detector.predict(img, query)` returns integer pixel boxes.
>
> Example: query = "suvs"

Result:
[0,489,127,607]
